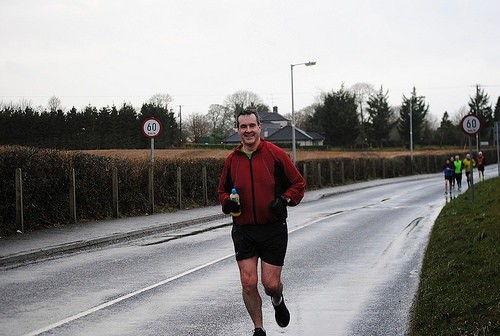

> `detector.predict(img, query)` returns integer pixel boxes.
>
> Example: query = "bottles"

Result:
[230,189,241,216]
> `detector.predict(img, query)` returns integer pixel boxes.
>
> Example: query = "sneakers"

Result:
[252,328,267,336]
[271,296,291,328]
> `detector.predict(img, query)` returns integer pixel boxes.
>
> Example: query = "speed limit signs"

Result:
[141,118,162,138]
[462,114,481,135]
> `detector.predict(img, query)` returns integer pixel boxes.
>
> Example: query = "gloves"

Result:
[222,200,240,215]
[269,197,287,218]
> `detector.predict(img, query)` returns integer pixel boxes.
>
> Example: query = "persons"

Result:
[449,156,456,190]
[443,158,455,194]
[217,110,309,336]
[453,154,465,191]
[462,153,477,188]
[476,152,485,181]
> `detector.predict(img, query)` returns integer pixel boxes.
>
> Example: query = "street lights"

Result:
[290,61,316,165]
[408,95,426,174]
